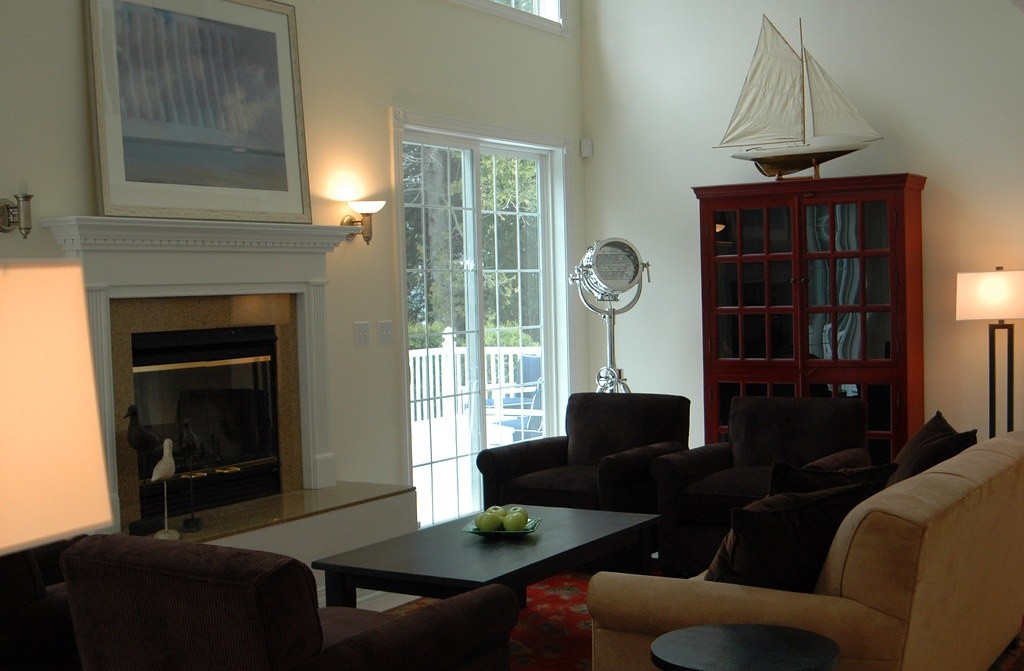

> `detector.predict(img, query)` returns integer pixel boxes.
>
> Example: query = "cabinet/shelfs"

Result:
[691,174,926,460]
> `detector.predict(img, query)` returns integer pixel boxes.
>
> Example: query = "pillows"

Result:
[701,411,980,592]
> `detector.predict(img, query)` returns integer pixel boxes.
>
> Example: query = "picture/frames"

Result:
[84,0,312,221]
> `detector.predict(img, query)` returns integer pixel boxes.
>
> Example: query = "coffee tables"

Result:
[310,504,659,614]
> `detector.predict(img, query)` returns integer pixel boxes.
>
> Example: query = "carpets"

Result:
[382,574,598,670]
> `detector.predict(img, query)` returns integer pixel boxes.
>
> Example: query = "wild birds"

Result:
[151,439,176,482]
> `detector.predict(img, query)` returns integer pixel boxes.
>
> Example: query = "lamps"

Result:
[0,183,35,240]
[339,199,388,246]
[568,236,651,393]
[955,267,1024,444]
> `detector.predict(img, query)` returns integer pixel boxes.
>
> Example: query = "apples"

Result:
[475,504,529,531]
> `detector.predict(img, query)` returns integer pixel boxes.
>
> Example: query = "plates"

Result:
[461,517,544,537]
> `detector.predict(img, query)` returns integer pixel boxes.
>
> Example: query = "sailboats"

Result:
[710,10,886,180]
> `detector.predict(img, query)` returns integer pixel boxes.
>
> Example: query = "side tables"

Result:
[649,622,840,670]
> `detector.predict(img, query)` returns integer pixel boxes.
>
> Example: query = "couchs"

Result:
[652,398,875,565]
[588,427,1024,671]
[477,390,690,574]
[0,518,520,670]
